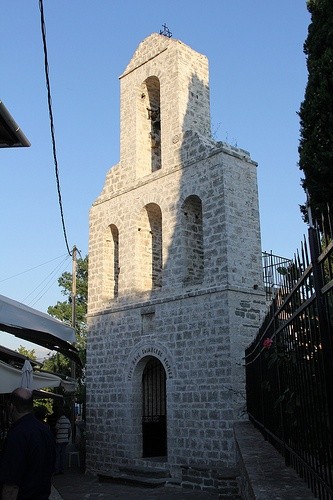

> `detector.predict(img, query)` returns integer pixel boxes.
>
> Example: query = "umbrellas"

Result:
[21,356,33,389]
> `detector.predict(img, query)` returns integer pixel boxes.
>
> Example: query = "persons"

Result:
[52,408,71,475]
[0,386,56,500]
